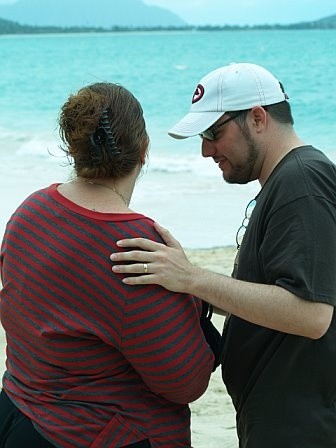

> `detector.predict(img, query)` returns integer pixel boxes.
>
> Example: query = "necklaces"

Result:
[77,178,127,209]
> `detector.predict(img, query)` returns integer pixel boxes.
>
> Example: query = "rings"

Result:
[144,263,148,273]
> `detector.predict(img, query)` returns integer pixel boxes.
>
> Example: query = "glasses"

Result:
[198,106,270,141]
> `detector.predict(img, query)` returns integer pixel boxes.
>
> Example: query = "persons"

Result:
[0,82,216,448]
[109,62,336,448]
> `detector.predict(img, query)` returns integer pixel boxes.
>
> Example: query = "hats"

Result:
[167,63,290,139]
